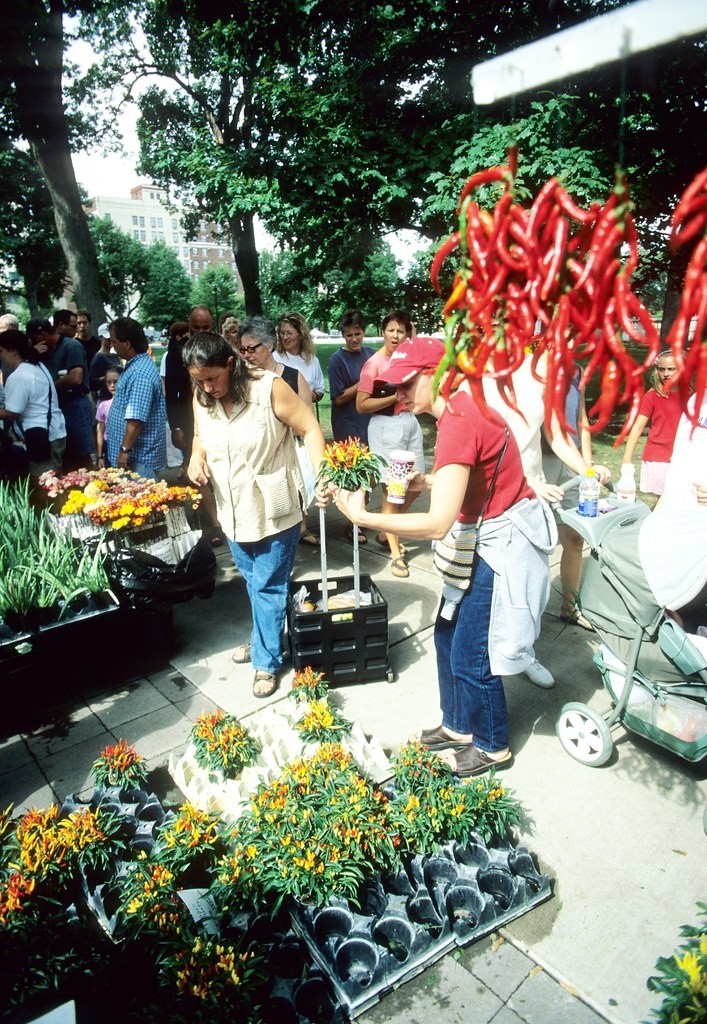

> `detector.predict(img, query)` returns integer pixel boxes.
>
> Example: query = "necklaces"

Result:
[222,396,235,404]
[273,360,277,373]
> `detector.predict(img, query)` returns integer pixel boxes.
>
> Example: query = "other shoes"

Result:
[561,604,596,632]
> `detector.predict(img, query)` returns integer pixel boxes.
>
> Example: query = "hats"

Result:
[374,337,446,388]
[98,323,112,339]
[26,317,52,336]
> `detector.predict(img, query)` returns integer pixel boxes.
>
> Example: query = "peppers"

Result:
[0,664,506,999]
[671,930,707,1024]
[321,435,372,471]
[430,139,707,450]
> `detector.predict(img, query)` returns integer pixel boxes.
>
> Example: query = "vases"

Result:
[348,482,371,506]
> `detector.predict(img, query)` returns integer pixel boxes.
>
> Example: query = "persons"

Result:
[0,329,67,519]
[106,317,167,482]
[164,306,215,467]
[326,309,557,778]
[221,311,316,438]
[52,309,126,457]
[540,316,616,631]
[615,351,707,497]
[691,480,707,506]
[160,322,191,396]
[180,329,331,697]
[0,312,27,487]
[27,319,96,476]
[96,366,124,470]
[272,310,325,547]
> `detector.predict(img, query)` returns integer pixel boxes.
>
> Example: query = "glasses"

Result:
[240,342,265,354]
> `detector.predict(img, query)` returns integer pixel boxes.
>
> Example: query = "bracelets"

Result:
[174,428,181,430]
[97,455,104,460]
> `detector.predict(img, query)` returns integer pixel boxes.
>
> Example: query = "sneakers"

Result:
[524,659,557,688]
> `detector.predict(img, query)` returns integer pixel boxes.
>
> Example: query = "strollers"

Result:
[551,472,707,768]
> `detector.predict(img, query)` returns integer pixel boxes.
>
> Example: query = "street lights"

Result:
[212,287,219,333]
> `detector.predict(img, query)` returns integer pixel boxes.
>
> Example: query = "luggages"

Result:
[287,475,394,687]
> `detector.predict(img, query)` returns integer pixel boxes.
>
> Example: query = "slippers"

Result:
[416,723,474,749]
[392,557,410,578]
[203,525,227,548]
[375,536,408,554]
[440,748,515,777]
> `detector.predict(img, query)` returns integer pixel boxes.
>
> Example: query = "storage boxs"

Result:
[287,573,392,686]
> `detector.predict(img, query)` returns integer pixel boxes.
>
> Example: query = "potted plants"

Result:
[0,474,150,742]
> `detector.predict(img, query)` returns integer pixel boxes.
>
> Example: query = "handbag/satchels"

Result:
[24,427,52,462]
[431,531,478,591]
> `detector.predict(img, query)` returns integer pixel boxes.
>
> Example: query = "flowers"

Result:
[0,669,527,1024]
[39,467,205,532]
[640,899,707,1024]
[312,434,388,498]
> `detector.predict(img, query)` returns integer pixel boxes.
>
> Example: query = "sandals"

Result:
[348,528,368,544]
[300,530,327,547]
[233,643,252,662]
[253,668,280,697]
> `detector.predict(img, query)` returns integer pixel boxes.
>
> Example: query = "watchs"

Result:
[311,390,319,403]
[120,446,133,454]
